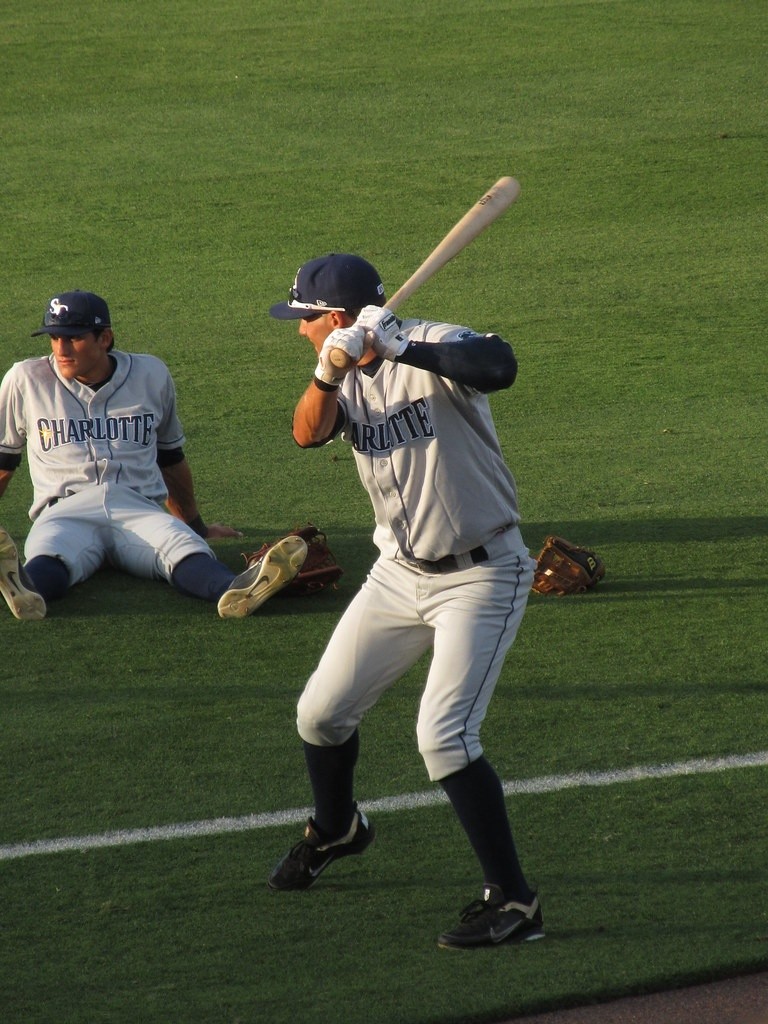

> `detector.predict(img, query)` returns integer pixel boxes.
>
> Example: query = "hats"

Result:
[269,253,385,320]
[31,289,111,338]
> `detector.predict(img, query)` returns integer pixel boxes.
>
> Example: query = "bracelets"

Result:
[187,514,208,538]
[314,377,339,392]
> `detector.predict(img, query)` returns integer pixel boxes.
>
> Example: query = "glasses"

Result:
[42,311,110,328]
[288,286,346,312]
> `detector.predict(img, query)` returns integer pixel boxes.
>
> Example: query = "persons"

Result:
[0,289,307,621]
[267,253,546,950]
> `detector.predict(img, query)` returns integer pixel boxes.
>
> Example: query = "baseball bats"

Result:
[332,177,521,367]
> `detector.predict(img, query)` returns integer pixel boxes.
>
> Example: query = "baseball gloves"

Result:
[241,521,344,586]
[531,536,607,599]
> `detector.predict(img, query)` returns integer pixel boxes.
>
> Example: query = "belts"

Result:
[414,546,489,574]
[48,498,58,507]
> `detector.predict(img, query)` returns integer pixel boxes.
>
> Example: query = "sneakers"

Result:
[267,801,375,891]
[439,884,546,949]
[217,535,309,619]
[0,526,48,621]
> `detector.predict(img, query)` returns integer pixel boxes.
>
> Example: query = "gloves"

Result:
[314,324,375,385]
[357,305,409,362]
